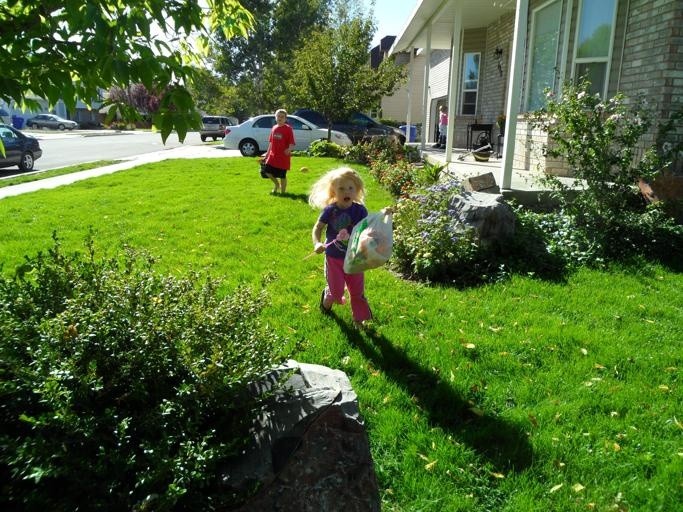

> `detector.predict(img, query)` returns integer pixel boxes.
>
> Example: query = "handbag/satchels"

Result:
[261,159,268,178]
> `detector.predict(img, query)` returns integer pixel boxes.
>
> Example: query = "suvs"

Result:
[291,107,406,147]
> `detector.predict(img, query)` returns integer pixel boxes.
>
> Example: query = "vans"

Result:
[199,116,233,142]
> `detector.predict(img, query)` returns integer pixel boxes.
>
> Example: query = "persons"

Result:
[432,105,448,150]
[261,109,294,195]
[307,167,395,325]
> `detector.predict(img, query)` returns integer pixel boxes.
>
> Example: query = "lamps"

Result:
[492,46,502,60]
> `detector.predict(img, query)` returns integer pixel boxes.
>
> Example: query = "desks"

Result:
[464,122,493,153]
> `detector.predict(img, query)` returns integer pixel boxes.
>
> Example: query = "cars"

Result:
[26,113,78,131]
[0,123,43,172]
[223,114,353,158]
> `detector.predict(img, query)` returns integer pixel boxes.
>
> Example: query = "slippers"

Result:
[319,289,331,313]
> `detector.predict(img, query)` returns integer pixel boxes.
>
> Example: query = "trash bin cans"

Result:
[399,125,416,142]
